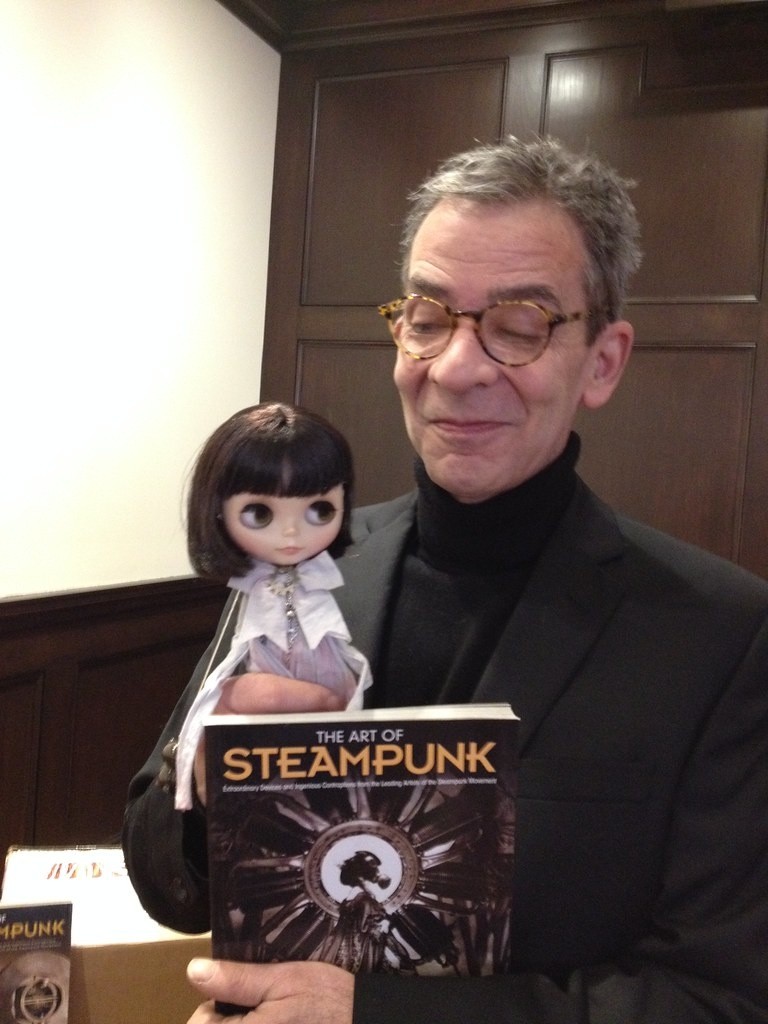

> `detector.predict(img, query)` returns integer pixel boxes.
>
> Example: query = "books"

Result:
[0,902,72,1024]
[201,701,511,1022]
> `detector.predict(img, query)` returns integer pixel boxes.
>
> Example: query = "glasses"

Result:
[378,294,591,367]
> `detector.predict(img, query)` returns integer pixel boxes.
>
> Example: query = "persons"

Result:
[125,130,768,1024]
[188,401,375,713]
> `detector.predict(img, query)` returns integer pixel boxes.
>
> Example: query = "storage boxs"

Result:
[0,847,214,1024]
